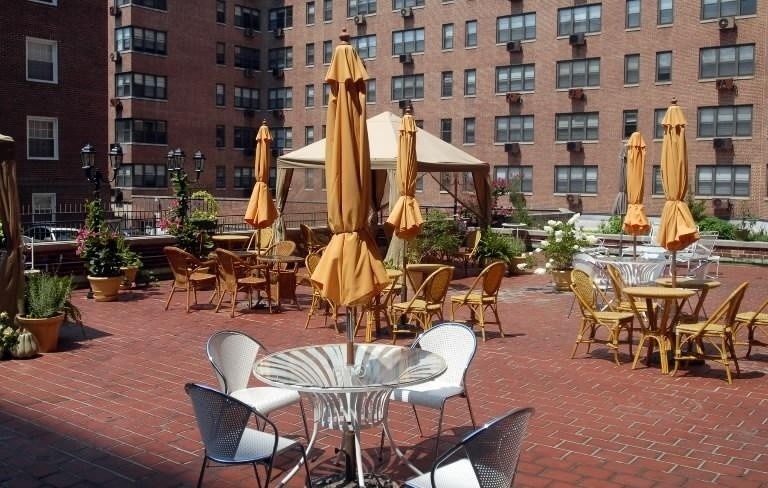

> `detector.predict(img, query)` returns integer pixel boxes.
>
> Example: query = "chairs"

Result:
[300,220,534,342]
[184,382,314,488]
[397,407,536,487]
[203,329,314,469]
[568,224,768,387]
[373,321,481,467]
[162,233,301,315]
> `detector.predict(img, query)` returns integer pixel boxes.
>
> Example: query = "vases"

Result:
[85,273,121,301]
[550,268,577,290]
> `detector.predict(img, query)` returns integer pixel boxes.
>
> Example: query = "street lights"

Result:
[163,148,208,234]
[76,143,126,237]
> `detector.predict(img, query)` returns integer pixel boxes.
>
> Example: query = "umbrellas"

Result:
[388,99,426,324]
[623,132,651,260]
[311,27,393,364]
[657,97,701,290]
[613,137,630,256]
[250,120,278,298]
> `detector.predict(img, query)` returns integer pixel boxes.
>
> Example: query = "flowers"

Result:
[75,197,125,276]
[516,212,601,276]
[156,197,187,232]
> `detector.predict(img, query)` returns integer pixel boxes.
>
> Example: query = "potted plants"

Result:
[12,270,87,353]
[733,200,756,239]
[120,244,144,284]
[187,190,218,231]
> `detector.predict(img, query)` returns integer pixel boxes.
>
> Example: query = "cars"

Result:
[25,226,90,244]
[117,225,151,237]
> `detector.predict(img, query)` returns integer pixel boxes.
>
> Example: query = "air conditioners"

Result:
[504,39,525,203]
[242,28,286,160]
[711,17,737,209]
[566,32,592,204]
[398,7,415,112]
[352,7,366,110]
[108,6,123,153]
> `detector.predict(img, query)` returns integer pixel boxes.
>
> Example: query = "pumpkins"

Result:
[9,327,40,359]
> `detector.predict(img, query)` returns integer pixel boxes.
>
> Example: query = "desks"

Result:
[252,343,447,487]
